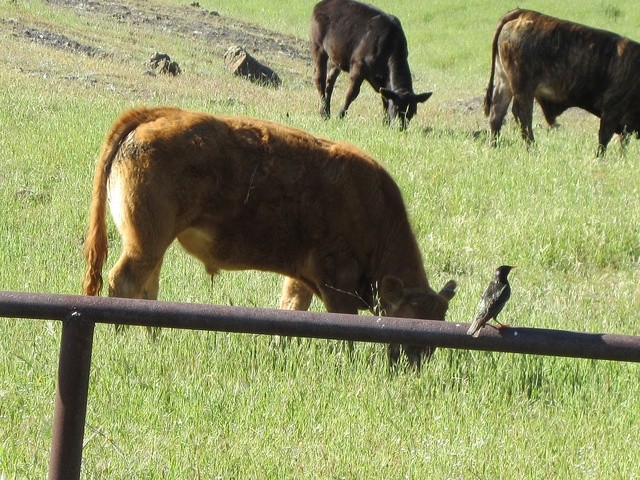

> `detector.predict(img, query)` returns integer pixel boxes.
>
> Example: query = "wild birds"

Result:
[467,266,516,337]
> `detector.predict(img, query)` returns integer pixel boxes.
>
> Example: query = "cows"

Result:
[311,0,433,130]
[483,7,640,158]
[80,106,456,374]
